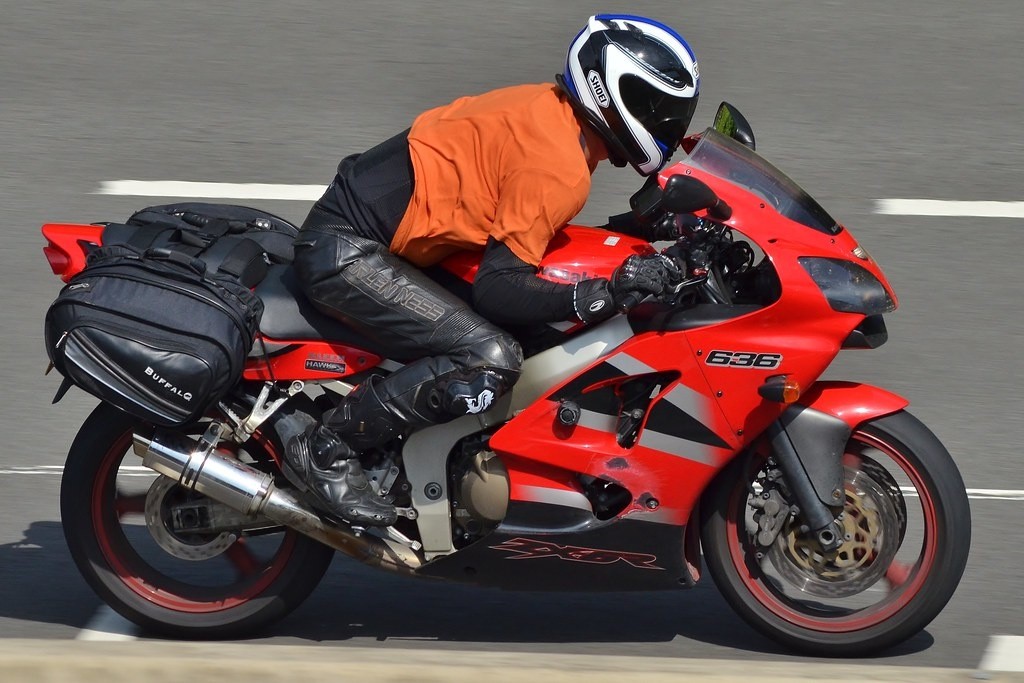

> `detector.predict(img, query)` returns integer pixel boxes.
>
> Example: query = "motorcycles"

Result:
[42,100,973,658]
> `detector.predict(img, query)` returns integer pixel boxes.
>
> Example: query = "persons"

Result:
[284,11,699,529]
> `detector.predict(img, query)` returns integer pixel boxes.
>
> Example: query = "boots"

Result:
[284,373,399,526]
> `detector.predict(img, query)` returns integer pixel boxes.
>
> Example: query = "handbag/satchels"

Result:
[44,241,265,432]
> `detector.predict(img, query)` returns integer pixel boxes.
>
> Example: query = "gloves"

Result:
[573,254,682,325]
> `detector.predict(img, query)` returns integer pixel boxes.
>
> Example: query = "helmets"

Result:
[556,15,699,178]
